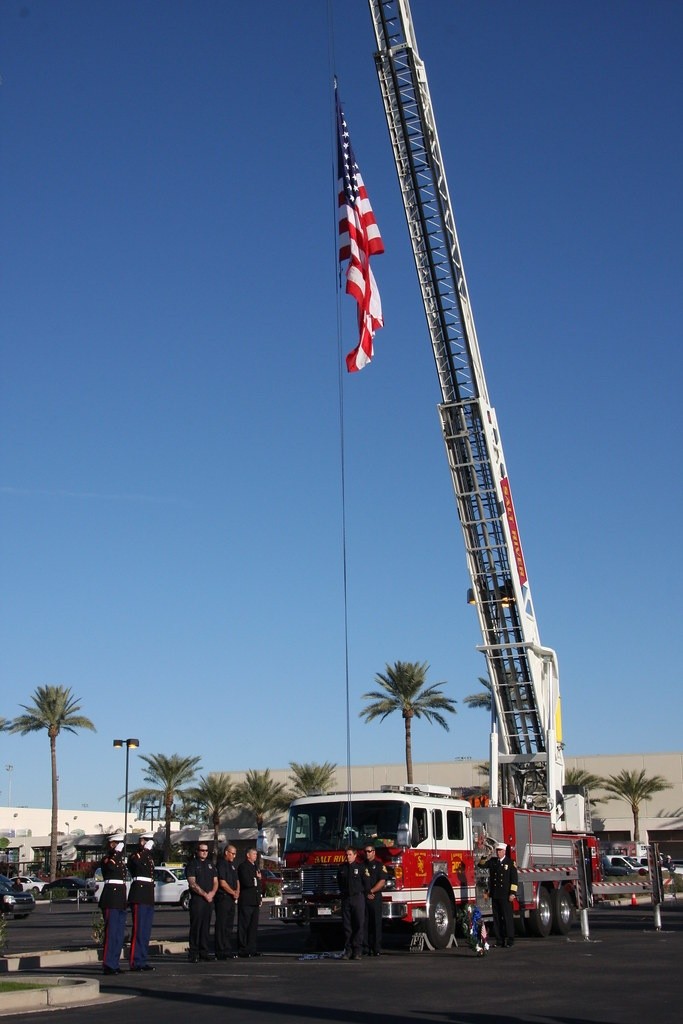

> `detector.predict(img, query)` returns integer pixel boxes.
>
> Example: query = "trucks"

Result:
[602,842,663,860]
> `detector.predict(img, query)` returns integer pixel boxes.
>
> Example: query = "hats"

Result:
[667,854,672,859]
[494,842,508,851]
[106,833,127,844]
[137,830,155,841]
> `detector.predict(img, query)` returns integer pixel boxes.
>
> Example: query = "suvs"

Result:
[604,854,649,874]
[94,867,191,911]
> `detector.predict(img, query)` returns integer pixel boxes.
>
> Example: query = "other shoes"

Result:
[238,951,263,958]
[130,964,154,971]
[362,952,381,956]
[340,952,353,960]
[103,967,127,975]
[188,953,212,963]
[354,953,362,960]
[215,953,238,960]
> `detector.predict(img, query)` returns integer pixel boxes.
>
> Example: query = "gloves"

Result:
[143,840,154,851]
[114,842,124,852]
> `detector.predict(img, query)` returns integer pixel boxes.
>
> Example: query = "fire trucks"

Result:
[271,1,675,950]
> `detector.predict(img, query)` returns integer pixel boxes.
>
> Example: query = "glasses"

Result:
[227,851,235,855]
[198,850,208,852]
[364,850,374,854]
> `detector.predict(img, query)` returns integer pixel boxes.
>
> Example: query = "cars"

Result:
[600,853,633,876]
[44,878,94,896]
[9,876,49,893]
[0,875,37,920]
[260,870,285,894]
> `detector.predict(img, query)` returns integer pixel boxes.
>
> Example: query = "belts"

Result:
[104,879,125,884]
[133,876,154,883]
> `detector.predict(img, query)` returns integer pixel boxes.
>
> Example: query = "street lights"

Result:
[113,739,140,832]
[6,764,14,807]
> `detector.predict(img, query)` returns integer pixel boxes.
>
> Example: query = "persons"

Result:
[359,845,388,956]
[214,845,241,959]
[336,846,371,960]
[663,855,677,873]
[12,879,23,892]
[126,830,156,972]
[236,848,264,958]
[184,844,218,962]
[477,843,518,948]
[98,833,129,975]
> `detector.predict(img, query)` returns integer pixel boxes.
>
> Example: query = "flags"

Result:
[334,77,387,372]
